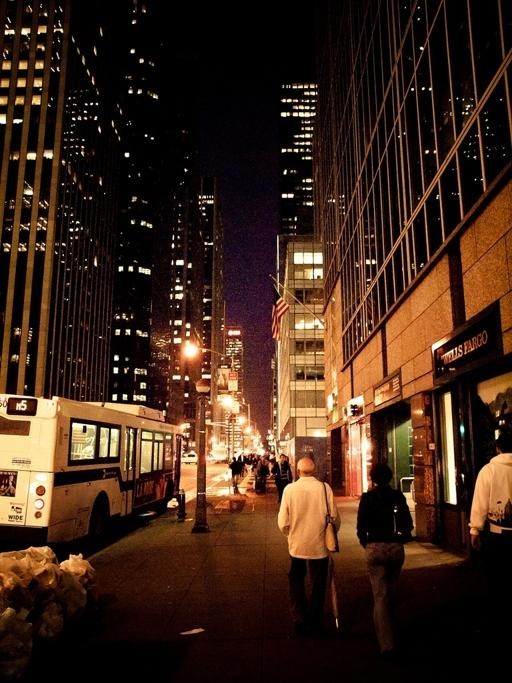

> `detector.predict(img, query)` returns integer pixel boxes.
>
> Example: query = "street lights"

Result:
[186,346,258,534]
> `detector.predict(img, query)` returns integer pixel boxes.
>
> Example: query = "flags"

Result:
[272,282,291,340]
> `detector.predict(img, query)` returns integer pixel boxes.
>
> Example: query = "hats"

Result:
[496,430,512,452]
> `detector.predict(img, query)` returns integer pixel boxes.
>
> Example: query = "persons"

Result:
[468,431,512,552]
[229,452,293,506]
[356,462,414,653]
[278,457,341,626]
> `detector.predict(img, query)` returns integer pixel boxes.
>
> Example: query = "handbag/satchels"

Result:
[325,522,339,551]
[393,507,404,536]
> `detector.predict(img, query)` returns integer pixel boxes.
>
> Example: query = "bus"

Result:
[0,393,185,544]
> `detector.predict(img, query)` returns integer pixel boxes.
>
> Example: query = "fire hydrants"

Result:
[176,489,187,523]
